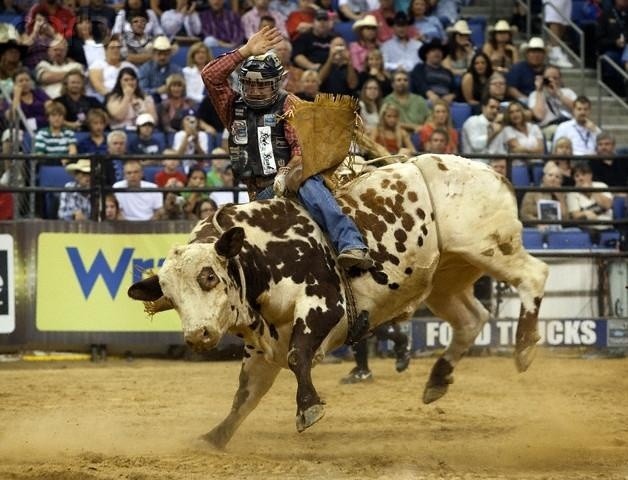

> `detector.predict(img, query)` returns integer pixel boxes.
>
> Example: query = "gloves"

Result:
[272,166,292,199]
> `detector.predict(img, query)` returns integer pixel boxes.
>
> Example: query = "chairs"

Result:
[1,0,628,251]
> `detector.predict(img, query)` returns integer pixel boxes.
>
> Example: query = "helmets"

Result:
[237,48,283,110]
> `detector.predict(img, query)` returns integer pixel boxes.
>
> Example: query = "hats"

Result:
[518,37,552,54]
[313,8,329,19]
[1,39,29,61]
[352,12,414,33]
[418,37,451,60]
[445,20,520,38]
[145,35,179,57]
[64,159,92,173]
[181,107,197,116]
[135,112,155,127]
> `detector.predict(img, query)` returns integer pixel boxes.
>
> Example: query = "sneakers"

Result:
[336,247,373,270]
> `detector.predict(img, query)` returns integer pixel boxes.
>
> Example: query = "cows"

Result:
[127,131,550,450]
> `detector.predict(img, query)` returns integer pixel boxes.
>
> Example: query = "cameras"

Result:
[543,77,550,85]
[335,44,345,51]
